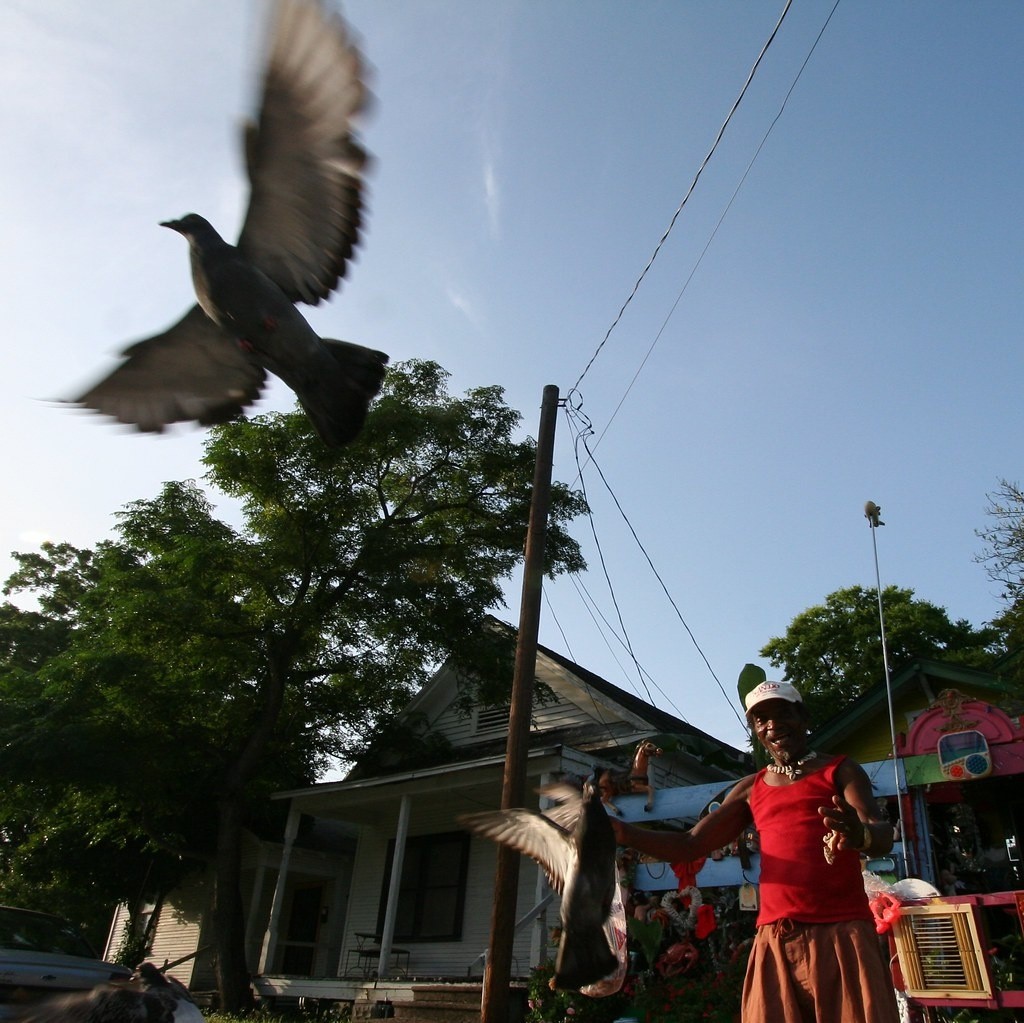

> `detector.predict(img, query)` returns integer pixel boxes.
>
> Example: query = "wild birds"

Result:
[38,3,393,453]
[456,765,621,993]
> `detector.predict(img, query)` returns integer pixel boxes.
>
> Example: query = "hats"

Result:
[745,681,802,717]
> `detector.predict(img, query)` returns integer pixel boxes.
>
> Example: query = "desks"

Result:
[344,933,410,980]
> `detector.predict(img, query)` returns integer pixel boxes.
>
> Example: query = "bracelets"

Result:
[863,825,873,850]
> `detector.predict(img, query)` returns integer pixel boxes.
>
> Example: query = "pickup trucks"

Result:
[0,904,134,1008]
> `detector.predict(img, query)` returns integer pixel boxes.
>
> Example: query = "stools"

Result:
[390,947,409,978]
[361,950,381,977]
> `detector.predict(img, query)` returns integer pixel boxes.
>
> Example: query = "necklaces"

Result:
[767,750,819,782]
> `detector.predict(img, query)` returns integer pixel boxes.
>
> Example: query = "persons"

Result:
[608,681,900,1023]
